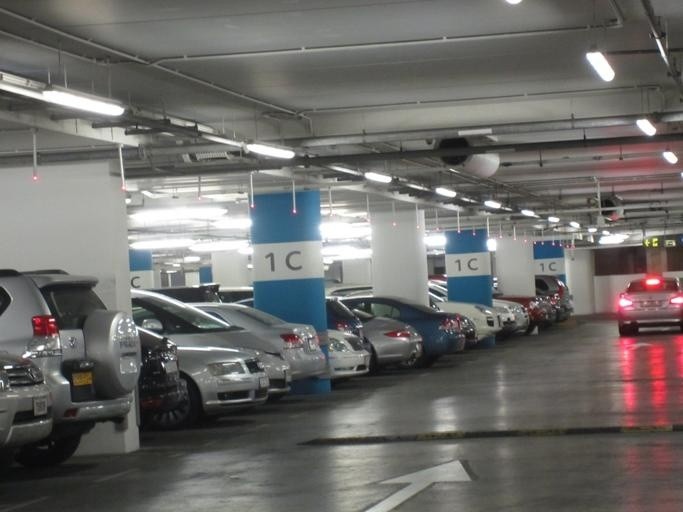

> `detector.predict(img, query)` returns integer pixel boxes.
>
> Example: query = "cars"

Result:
[617,276,683,337]
[131,301,328,382]
[329,272,576,379]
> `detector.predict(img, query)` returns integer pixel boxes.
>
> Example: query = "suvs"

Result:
[0,267,143,470]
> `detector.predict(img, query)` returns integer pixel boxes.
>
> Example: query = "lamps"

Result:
[40,40,296,160]
[324,147,502,209]
[587,0,679,164]
